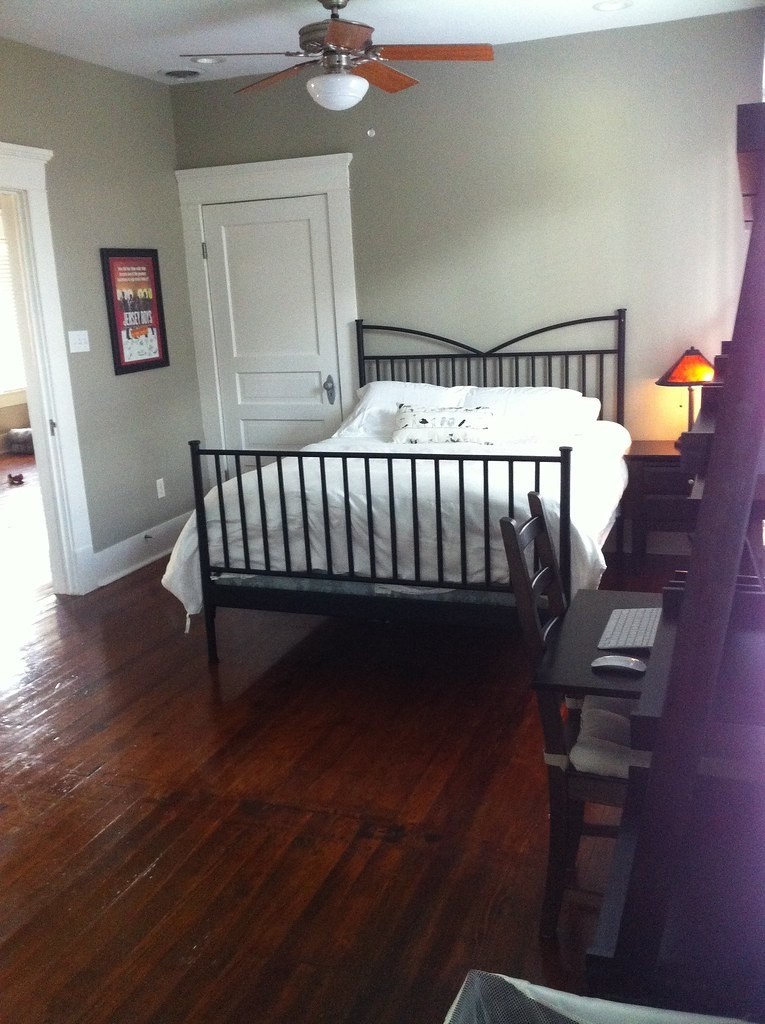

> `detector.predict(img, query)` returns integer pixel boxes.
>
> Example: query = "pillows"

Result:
[331,377,603,444]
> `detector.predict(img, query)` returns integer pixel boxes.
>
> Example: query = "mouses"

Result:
[591,655,647,676]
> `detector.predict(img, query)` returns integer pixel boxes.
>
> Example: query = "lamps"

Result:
[654,346,726,449]
[305,55,370,111]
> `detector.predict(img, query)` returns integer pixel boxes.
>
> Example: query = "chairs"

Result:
[500,491,640,949]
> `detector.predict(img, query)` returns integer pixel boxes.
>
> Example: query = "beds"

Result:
[189,309,632,668]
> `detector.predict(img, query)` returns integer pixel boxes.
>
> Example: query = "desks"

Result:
[531,587,681,950]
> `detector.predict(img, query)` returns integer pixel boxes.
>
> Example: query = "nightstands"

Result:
[622,440,697,576]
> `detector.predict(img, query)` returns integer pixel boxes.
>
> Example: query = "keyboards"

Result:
[597,608,661,650]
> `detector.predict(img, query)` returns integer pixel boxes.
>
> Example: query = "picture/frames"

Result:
[99,246,171,375]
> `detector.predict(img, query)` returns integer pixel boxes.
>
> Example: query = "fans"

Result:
[178,0,495,95]
